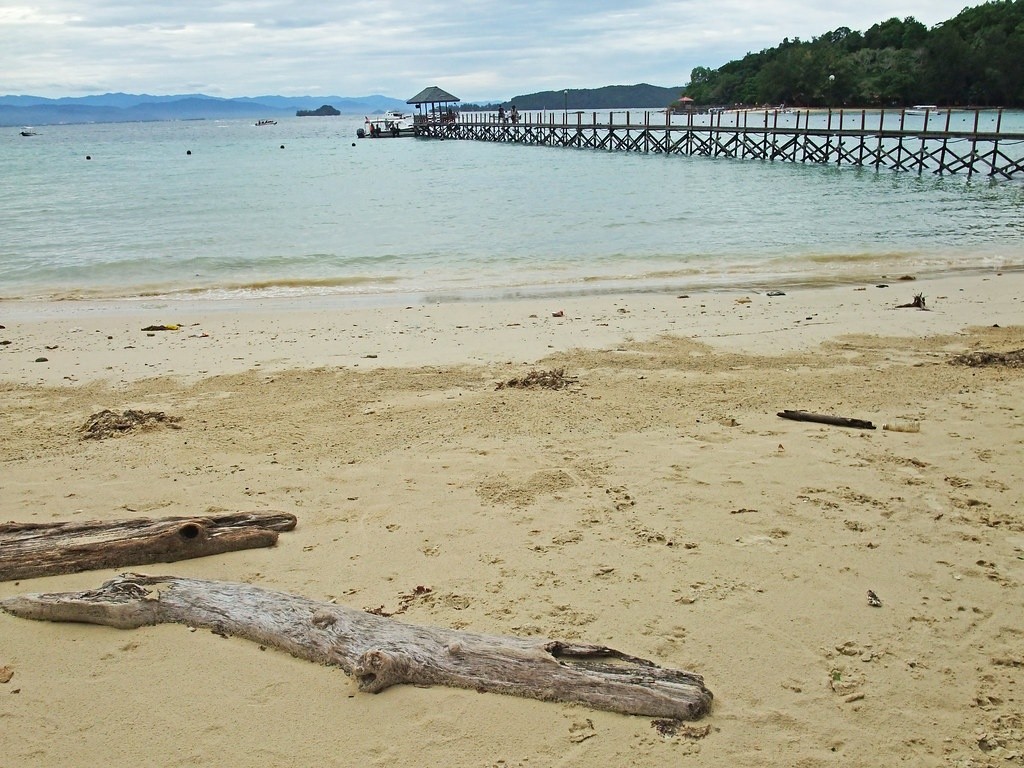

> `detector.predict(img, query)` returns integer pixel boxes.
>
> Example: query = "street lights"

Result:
[828,75,835,112]
[563,90,568,124]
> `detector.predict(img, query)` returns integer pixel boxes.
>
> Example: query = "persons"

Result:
[504,109,509,124]
[509,105,520,125]
[497,104,506,124]
[368,123,399,138]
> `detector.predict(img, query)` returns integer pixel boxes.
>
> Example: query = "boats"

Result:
[20,126,42,136]
[357,117,419,138]
[904,104,940,116]
[254,120,278,127]
[369,111,410,122]
